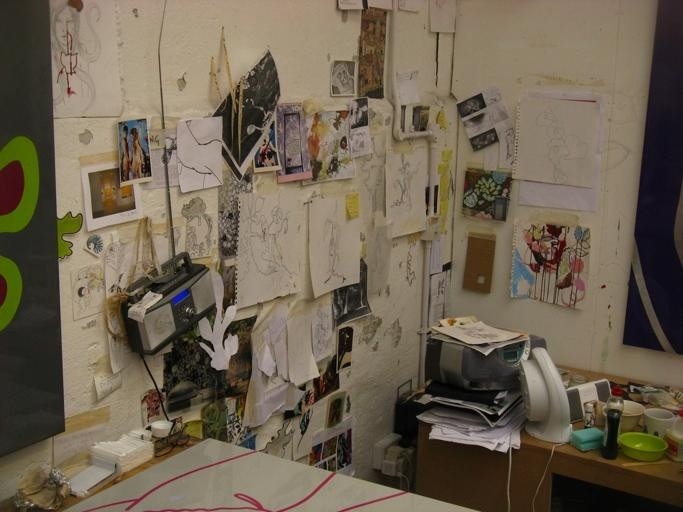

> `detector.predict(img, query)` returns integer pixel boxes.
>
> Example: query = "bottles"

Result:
[603,396,624,460]
[585,403,594,428]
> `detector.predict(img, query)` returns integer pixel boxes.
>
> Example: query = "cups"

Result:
[152,420,173,438]
[646,408,675,438]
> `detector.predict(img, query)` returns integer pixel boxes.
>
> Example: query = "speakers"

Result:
[566,379,612,424]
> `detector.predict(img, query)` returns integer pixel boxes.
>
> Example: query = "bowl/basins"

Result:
[619,431,668,462]
[602,399,644,430]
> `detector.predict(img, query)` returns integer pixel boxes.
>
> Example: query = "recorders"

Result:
[121,252,216,355]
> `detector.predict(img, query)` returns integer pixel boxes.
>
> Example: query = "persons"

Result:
[130,128,145,177]
[121,125,131,180]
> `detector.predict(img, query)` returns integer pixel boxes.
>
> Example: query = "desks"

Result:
[413,365,682,512]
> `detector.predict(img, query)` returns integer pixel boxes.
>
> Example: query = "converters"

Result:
[381,445,416,477]
